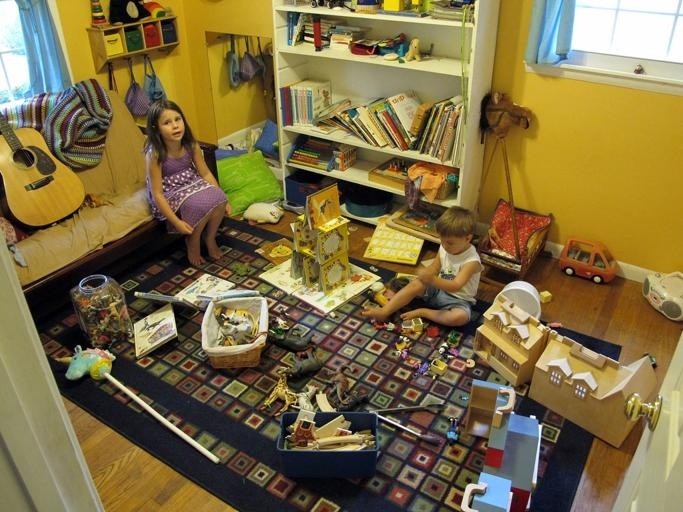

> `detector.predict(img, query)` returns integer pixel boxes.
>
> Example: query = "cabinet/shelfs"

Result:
[270,0,504,214]
[83,13,181,77]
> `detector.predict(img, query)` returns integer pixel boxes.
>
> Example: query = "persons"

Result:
[360,205,486,327]
[141,99,228,264]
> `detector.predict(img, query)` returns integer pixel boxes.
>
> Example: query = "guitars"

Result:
[0,114,86,229]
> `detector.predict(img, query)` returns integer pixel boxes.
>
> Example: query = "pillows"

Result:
[214,118,282,225]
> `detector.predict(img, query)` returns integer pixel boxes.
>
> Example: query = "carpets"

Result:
[20,212,624,512]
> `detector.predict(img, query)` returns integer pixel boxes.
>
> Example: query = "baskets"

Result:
[201,296,268,369]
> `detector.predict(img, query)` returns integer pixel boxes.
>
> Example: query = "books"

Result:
[287,12,372,50]
[280,78,466,168]
[289,182,351,296]
[132,273,236,360]
[429,0,474,23]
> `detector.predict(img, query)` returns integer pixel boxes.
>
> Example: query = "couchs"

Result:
[0,90,230,296]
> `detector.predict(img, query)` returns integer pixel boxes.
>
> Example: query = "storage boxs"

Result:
[285,172,324,207]
[271,404,381,482]
